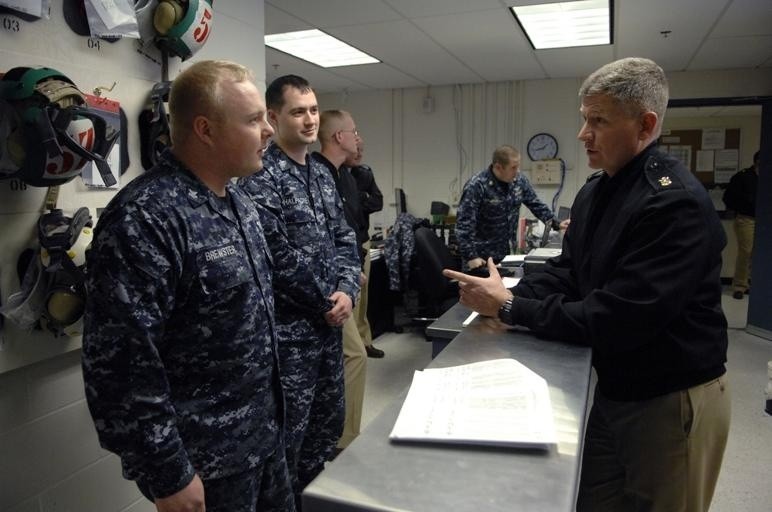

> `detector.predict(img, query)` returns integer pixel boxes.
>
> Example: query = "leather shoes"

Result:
[366,344,385,359]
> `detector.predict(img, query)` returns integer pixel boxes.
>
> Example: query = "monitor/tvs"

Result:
[395,188,407,218]
[558,206,570,221]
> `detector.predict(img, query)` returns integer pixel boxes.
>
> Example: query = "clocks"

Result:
[527,132,558,161]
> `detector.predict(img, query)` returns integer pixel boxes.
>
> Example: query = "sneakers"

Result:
[733,288,751,300]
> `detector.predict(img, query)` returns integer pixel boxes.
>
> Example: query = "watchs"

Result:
[499,298,513,325]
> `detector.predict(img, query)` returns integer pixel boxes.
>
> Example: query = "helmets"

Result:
[0,65,116,190]
[19,205,98,339]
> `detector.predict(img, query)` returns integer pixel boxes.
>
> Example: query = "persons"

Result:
[311,110,367,462]
[443,58,732,512]
[339,149,368,286]
[722,149,761,300]
[348,134,385,359]
[453,144,571,273]
[236,72,363,500]
[80,59,301,512]
